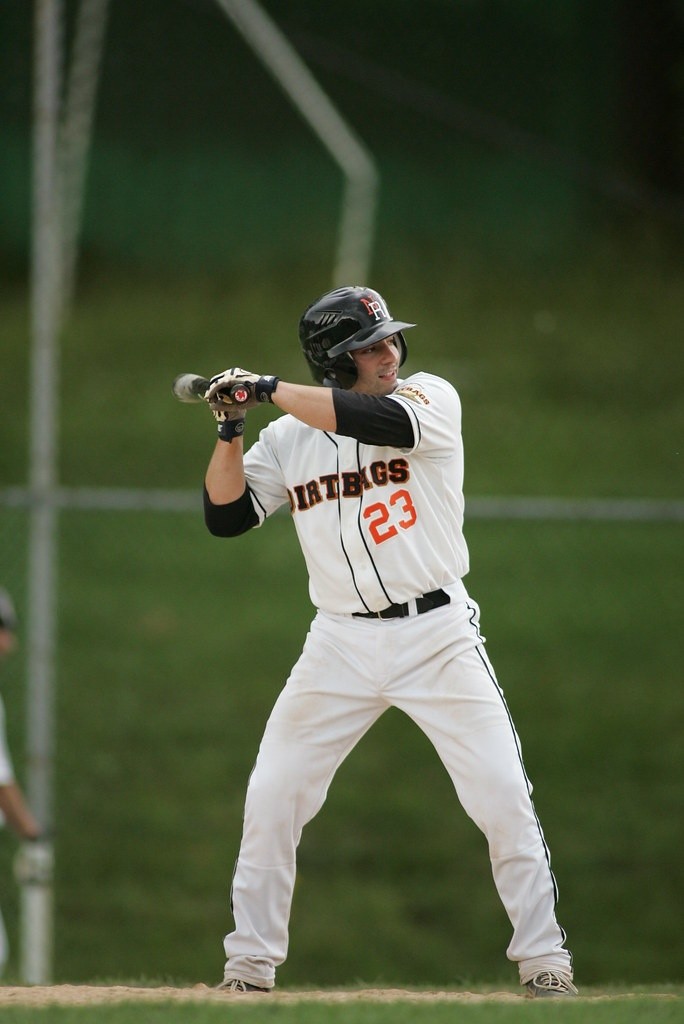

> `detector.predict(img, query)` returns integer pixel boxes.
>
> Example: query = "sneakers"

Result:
[194,979,270,994]
[527,971,578,1000]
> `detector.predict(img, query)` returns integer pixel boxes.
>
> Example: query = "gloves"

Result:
[208,403,247,444]
[204,368,281,411]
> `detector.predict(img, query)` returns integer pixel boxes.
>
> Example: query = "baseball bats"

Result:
[173,371,251,405]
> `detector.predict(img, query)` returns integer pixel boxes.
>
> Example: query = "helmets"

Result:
[299,286,417,391]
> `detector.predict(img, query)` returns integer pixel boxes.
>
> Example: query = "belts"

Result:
[352,588,450,621]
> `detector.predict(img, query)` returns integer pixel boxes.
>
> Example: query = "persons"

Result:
[199,287,581,996]
